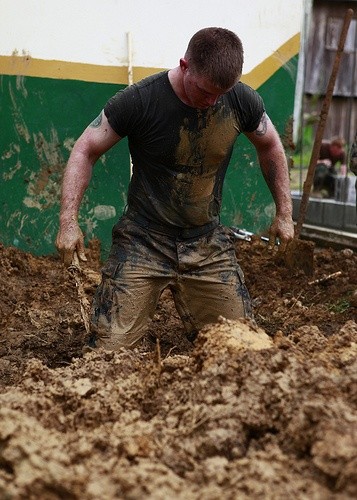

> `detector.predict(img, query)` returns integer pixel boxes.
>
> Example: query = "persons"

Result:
[316,135,347,190]
[53,25,297,356]
